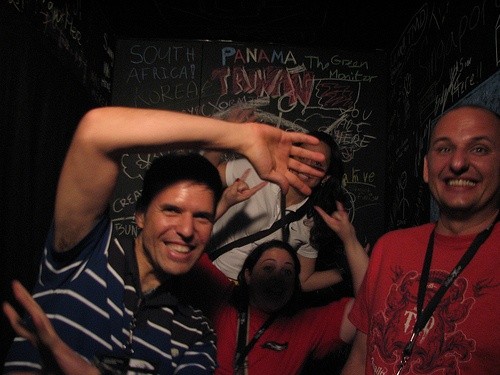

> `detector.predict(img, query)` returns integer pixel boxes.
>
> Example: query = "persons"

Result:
[191,168,369,375]
[3,107,324,375]
[341,105,500,375]
[202,107,369,291]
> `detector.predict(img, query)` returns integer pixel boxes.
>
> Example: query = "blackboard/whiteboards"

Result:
[107,1,500,306]
